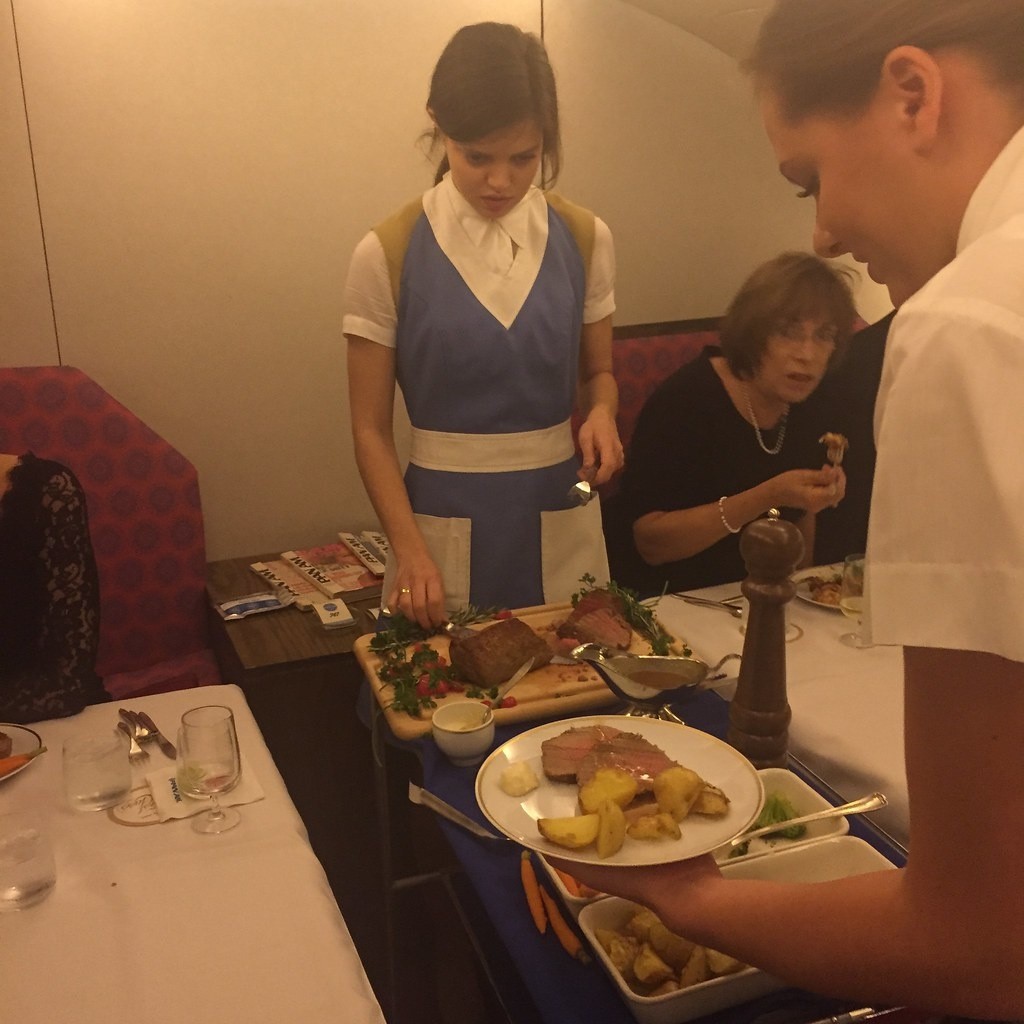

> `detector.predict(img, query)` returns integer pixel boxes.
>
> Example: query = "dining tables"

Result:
[0,686,384,1024]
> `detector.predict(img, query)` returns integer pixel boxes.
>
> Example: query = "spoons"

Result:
[460,657,536,730]
[118,707,159,742]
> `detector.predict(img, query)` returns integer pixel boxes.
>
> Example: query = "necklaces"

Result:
[742,377,787,455]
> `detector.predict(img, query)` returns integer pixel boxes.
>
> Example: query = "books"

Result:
[249,560,381,612]
[280,542,384,600]
[338,531,388,576]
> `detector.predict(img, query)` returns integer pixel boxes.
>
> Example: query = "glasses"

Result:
[780,318,839,347]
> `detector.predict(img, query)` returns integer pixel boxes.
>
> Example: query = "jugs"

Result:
[570,642,743,726]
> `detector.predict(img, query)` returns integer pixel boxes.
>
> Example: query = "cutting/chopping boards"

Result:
[352,601,721,741]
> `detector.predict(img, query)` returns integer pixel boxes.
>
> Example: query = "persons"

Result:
[613,250,863,601]
[0,450,114,725]
[344,21,626,631]
[540,0,1024,1024]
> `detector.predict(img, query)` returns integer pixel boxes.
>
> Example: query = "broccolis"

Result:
[725,793,808,857]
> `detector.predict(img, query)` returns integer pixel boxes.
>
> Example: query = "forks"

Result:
[827,445,844,508]
[116,722,151,760]
[566,458,601,507]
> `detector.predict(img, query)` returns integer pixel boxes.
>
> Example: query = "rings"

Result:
[400,589,411,593]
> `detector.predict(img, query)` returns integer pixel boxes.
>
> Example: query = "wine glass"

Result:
[839,553,876,648]
[181,705,243,835]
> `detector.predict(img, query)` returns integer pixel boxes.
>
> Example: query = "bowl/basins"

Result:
[535,768,902,1024]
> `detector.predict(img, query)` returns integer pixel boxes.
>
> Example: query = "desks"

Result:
[639,558,911,851]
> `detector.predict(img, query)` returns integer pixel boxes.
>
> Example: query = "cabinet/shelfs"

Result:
[355,591,969,1024]
[205,540,395,700]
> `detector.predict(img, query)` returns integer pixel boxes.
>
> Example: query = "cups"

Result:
[741,598,791,635]
[430,701,496,768]
[0,811,57,914]
[62,728,132,812]
[177,727,210,800]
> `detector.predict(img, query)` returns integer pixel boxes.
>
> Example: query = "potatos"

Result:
[497,758,726,858]
[593,905,745,999]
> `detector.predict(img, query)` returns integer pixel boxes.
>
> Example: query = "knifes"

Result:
[139,712,177,760]
[381,607,578,665]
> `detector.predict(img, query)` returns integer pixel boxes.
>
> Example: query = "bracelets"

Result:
[719,496,742,533]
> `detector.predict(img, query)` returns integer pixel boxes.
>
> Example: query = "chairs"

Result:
[572,316,873,500]
[0,366,224,698]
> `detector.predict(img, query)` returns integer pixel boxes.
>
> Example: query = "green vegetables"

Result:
[367,572,691,713]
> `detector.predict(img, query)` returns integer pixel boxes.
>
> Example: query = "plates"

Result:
[474,715,767,868]
[789,563,843,610]
[0,723,42,782]
